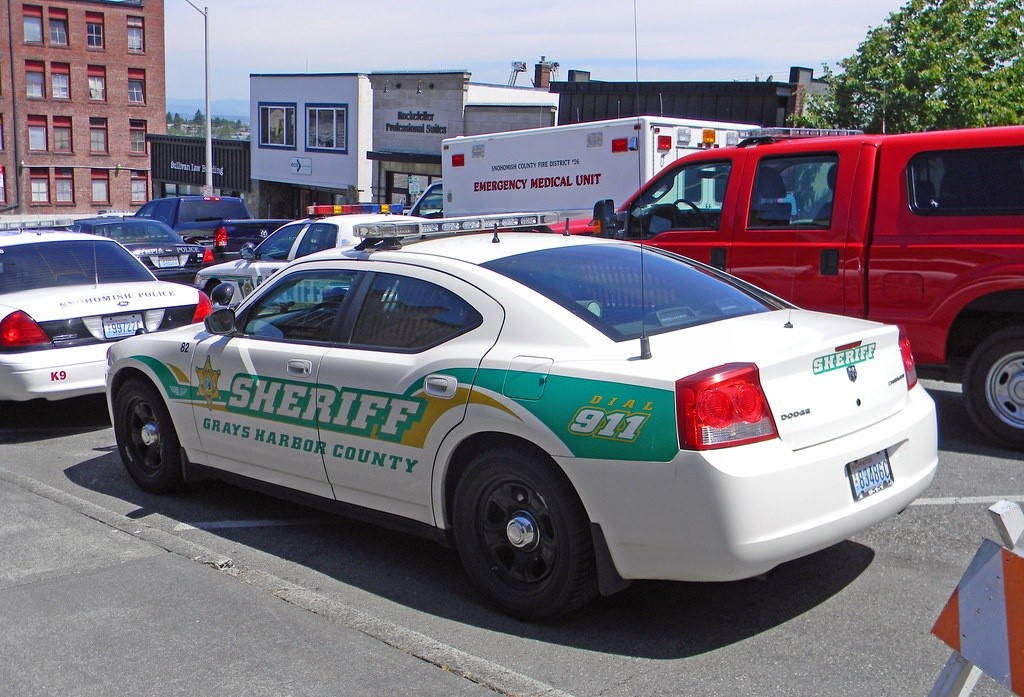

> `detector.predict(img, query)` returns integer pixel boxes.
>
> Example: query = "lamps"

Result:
[20,159,25,165]
[416,79,433,94]
[383,79,400,93]
[116,162,121,168]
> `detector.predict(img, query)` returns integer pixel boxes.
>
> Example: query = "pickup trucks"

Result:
[545,126,1022,449]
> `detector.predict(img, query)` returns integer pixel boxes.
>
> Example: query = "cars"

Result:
[1,197,937,621]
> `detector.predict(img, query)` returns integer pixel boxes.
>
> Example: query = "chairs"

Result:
[751,173,791,226]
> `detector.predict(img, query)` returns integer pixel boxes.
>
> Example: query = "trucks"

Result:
[405,115,760,226]
